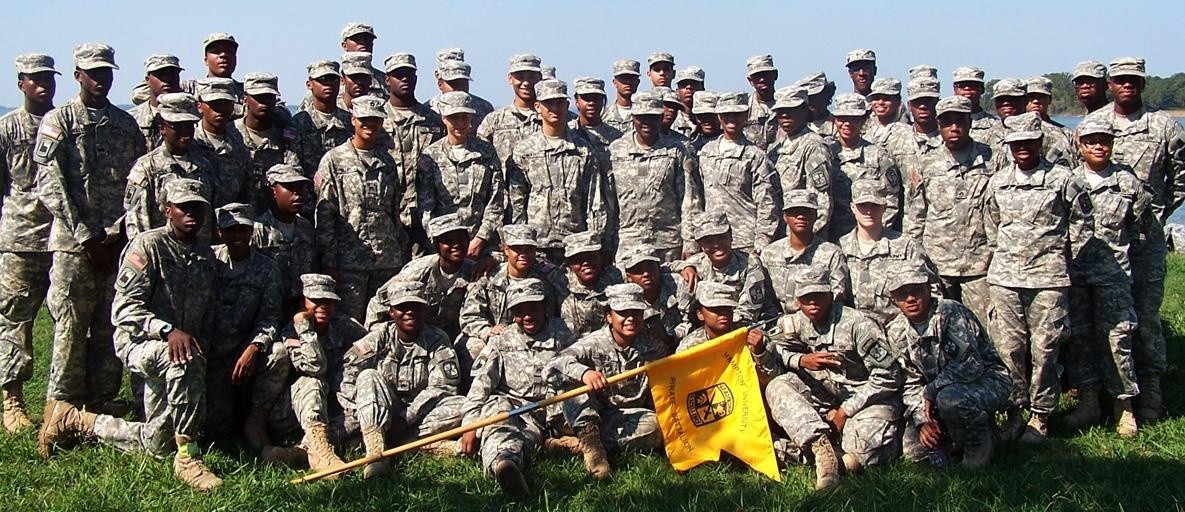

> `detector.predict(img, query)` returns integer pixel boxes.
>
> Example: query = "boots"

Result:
[491,454,533,504]
[300,420,352,480]
[1136,371,1163,423]
[359,425,391,483]
[1059,382,1104,429]
[957,429,997,474]
[171,431,226,493]
[543,435,584,461]
[1111,392,1139,439]
[244,406,272,451]
[1,379,38,438]
[810,432,840,496]
[39,398,98,461]
[996,405,1050,450]
[575,418,613,483]
[259,440,309,470]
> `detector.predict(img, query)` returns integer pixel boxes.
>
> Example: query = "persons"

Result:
[1,24,1184,498]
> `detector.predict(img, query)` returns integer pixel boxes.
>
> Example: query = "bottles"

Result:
[926,409,951,475]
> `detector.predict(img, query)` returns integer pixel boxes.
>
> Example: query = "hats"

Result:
[12,32,283,126]
[794,269,832,299]
[505,278,544,310]
[386,281,428,306]
[783,189,818,212]
[695,280,738,309]
[300,272,341,302]
[850,178,887,207]
[509,50,1149,143]
[691,208,730,240]
[304,21,478,120]
[562,230,602,258]
[164,163,312,230]
[622,243,661,270]
[886,258,929,290]
[604,282,649,312]
[498,224,539,249]
[425,213,468,242]
